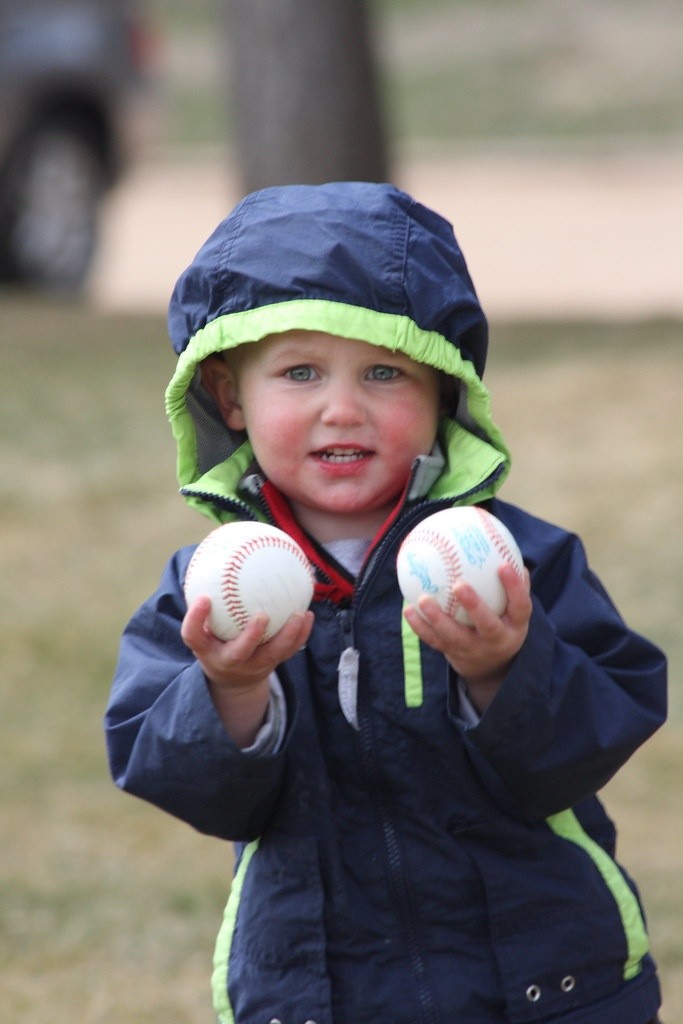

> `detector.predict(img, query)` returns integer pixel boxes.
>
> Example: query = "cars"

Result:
[0,1,156,299]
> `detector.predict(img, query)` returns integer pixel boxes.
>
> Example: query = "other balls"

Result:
[397,508,522,636]
[186,520,314,646]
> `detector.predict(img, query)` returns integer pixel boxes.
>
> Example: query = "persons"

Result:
[102,182,668,1024]
[0,0,164,309]
